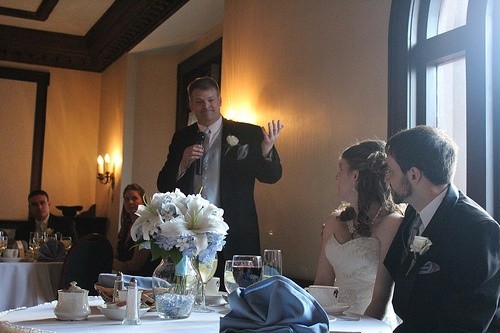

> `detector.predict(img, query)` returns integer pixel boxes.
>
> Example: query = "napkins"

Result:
[219,274,330,333]
[88,296,105,307]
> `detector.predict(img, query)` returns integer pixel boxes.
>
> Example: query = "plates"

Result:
[323,305,352,314]
[0,257,24,262]
[194,292,230,307]
[327,316,337,323]
[97,305,150,320]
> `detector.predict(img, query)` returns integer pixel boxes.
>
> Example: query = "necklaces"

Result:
[347,205,384,234]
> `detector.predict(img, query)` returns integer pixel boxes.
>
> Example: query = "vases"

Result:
[151,250,200,319]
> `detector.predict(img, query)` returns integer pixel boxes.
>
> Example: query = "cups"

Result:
[304,285,338,307]
[54,280,91,321]
[232,255,262,289]
[224,259,253,296]
[3,248,18,258]
[198,276,220,295]
[118,289,144,308]
[262,249,282,281]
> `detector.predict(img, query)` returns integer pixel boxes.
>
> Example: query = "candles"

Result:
[97,154,104,174]
[104,153,110,173]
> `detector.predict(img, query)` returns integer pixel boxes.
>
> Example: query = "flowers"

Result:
[130,188,229,270]
[405,235,433,276]
[223,135,239,156]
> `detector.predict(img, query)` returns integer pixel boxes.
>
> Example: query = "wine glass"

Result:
[0,231,72,263]
[192,250,214,313]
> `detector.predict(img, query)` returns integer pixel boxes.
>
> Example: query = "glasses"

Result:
[31,201,47,207]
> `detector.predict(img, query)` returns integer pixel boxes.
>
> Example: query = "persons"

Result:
[158,77,284,296]
[15,190,77,243]
[314,141,404,330]
[385,125,500,333]
[111,183,162,277]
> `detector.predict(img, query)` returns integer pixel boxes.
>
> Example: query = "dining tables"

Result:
[0,258,64,311]
[0,297,394,333]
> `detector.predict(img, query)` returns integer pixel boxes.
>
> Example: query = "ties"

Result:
[201,128,212,160]
[399,213,422,265]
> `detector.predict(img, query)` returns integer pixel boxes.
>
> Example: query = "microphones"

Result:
[196,132,205,175]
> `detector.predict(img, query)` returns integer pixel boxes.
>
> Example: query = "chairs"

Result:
[56,233,114,300]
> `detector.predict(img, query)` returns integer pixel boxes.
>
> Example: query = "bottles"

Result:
[122,276,140,325]
[112,271,124,309]
